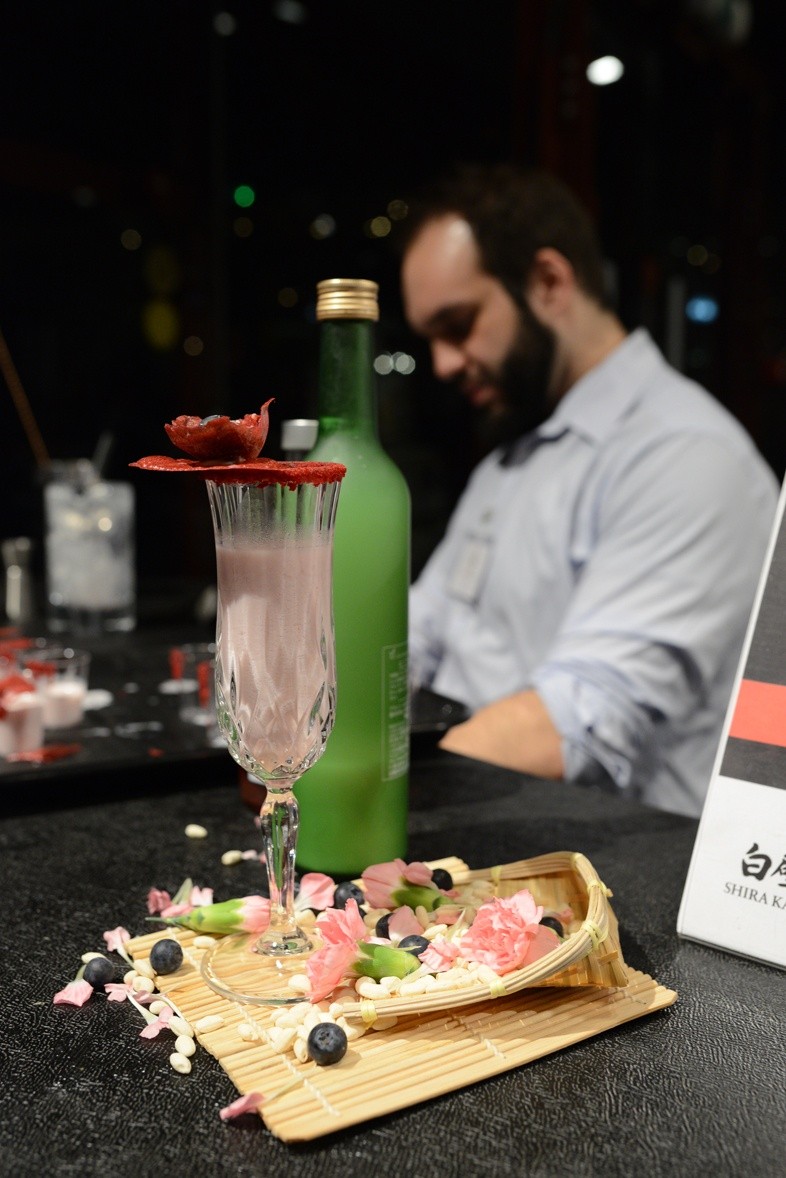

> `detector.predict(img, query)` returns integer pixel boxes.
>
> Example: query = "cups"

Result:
[0,634,94,760]
[170,643,236,750]
[45,476,135,634]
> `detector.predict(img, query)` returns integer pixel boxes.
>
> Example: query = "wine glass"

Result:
[199,463,348,1003]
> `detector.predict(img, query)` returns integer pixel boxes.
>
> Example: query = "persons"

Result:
[405,149,782,821]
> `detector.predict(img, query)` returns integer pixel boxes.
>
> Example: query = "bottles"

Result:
[270,278,410,875]
